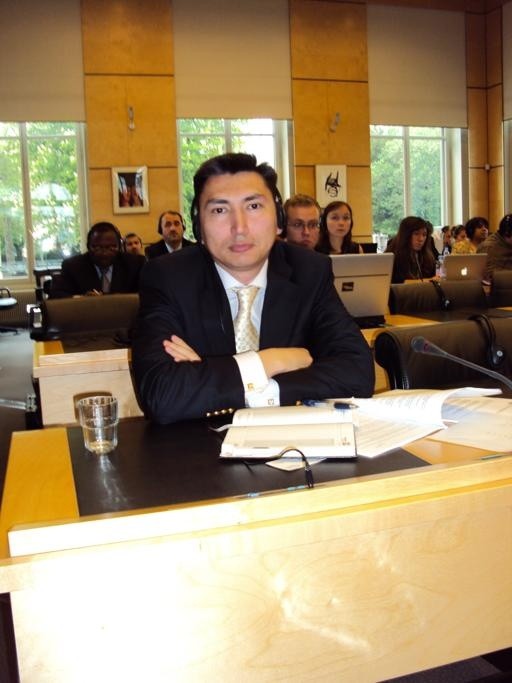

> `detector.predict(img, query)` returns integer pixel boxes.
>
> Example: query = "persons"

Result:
[131,152,375,426]
[280,196,363,256]
[60,211,194,297]
[384,214,512,300]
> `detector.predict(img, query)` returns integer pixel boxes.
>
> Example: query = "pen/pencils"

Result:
[93,289,100,295]
[303,399,359,409]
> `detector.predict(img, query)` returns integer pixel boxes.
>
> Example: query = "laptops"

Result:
[327,251,396,327]
[443,253,488,281]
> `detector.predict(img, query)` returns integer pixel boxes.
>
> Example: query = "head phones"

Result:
[157,212,186,234]
[188,187,286,239]
[88,222,124,249]
[429,280,454,312]
[468,312,507,369]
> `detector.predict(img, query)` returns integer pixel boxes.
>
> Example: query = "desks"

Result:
[0,395,512,683]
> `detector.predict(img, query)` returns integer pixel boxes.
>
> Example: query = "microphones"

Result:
[410,336,512,392]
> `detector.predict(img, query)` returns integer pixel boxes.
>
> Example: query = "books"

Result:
[220,385,503,460]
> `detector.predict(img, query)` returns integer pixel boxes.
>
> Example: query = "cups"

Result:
[78,395,120,454]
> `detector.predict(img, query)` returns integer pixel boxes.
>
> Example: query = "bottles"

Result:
[435,246,450,278]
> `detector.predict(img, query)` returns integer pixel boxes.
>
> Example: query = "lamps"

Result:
[329,111,341,132]
[127,106,136,131]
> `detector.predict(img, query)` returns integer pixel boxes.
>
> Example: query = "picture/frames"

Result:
[110,164,149,216]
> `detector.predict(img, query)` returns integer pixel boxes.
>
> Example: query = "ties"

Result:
[230,285,262,354]
[99,267,111,292]
